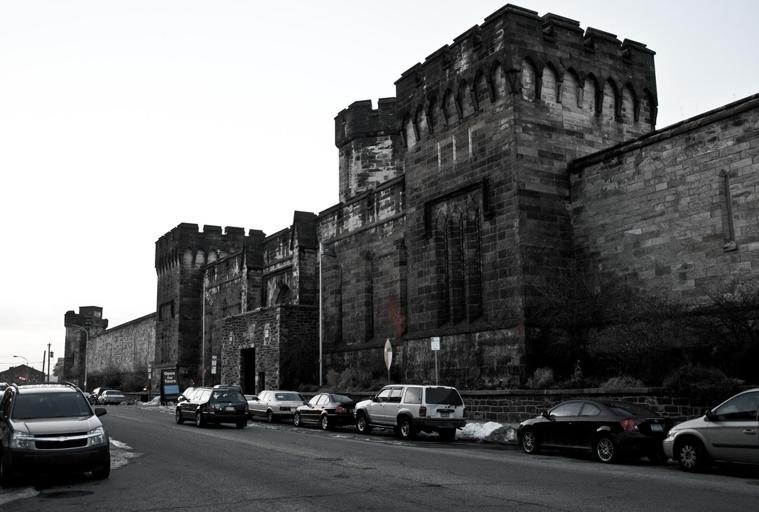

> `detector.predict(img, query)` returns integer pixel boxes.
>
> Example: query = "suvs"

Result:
[352,383,468,445]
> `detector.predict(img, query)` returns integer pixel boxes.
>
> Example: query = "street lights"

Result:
[13,355,28,384]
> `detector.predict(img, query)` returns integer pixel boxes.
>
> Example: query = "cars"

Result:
[517,399,672,463]
[292,392,355,430]
[174,383,306,429]
[662,387,759,476]
[1,379,128,478]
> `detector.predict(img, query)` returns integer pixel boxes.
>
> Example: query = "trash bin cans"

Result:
[141,394,148,402]
